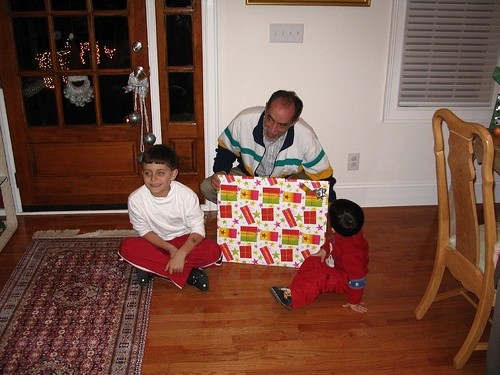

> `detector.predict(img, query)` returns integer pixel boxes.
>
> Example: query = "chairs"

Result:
[415,109,500,368]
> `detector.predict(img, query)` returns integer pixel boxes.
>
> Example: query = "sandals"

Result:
[187,267,209,291]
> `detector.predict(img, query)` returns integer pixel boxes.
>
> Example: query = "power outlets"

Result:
[348,153,360,171]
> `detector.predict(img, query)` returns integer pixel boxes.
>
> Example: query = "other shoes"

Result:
[137,270,153,284]
[270,287,293,310]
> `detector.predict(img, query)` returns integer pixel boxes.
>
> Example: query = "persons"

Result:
[200,90,336,207]
[117,144,223,290]
[268,197,370,314]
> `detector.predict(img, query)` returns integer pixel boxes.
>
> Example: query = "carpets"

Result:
[1,229,154,375]
